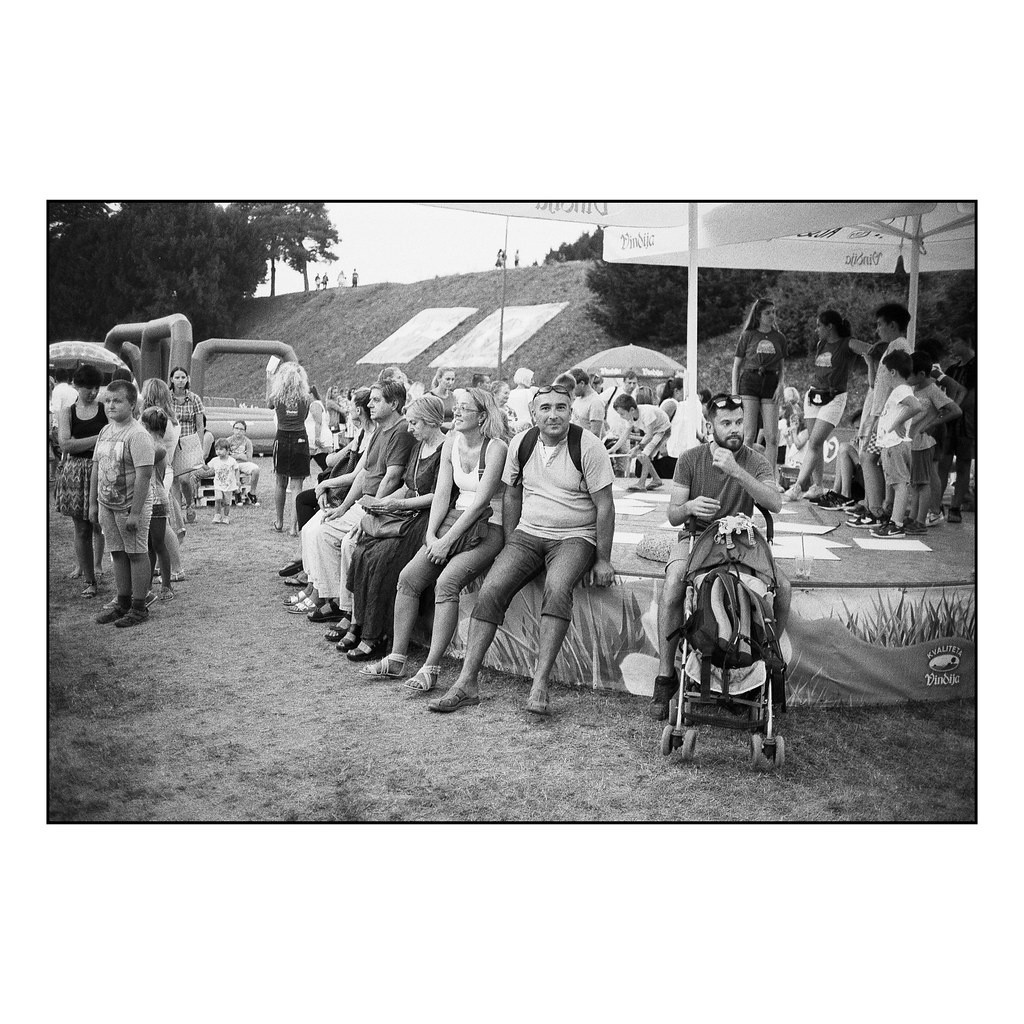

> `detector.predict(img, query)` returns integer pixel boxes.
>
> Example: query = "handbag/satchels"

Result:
[808,387,846,406]
[172,433,205,478]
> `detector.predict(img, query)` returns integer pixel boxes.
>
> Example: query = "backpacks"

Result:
[688,567,777,669]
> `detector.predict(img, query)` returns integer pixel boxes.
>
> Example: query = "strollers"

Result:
[660,503,793,770]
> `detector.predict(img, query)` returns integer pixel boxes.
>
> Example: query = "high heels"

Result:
[347,633,389,663]
[336,624,363,652]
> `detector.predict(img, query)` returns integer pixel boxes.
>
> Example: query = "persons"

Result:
[495,249,519,269]
[89,380,155,628]
[307,381,417,641]
[315,269,359,290]
[783,312,872,500]
[649,395,791,719]
[359,387,507,690]
[732,299,788,492]
[278,385,374,585]
[182,414,261,524]
[138,367,204,606]
[266,362,311,535]
[430,389,614,713]
[809,305,974,538]
[381,367,712,491]
[778,387,808,470]
[50,366,142,597]
[336,397,459,661]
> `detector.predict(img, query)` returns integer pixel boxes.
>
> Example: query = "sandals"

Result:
[68,508,195,627]
[403,664,440,690]
[324,614,352,642]
[358,653,407,678]
[307,599,348,622]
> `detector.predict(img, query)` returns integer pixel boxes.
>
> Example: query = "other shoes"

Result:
[212,514,222,523]
[234,492,244,506]
[247,492,260,507]
[222,516,229,524]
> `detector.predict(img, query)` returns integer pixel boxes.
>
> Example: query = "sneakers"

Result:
[648,669,680,719]
[624,483,962,538]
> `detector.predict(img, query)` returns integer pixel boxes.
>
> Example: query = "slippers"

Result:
[279,559,303,577]
[288,597,317,613]
[283,590,307,605]
[526,688,553,716]
[284,576,309,586]
[274,521,283,531]
[428,687,480,710]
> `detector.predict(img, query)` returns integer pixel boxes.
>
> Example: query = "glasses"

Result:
[234,427,244,431]
[532,385,571,402]
[452,404,481,413]
[707,395,742,412]
[755,298,773,308]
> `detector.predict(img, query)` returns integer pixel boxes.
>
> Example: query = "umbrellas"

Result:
[602,203,975,350]
[49,341,132,374]
[573,343,685,379]
[420,202,723,400]
